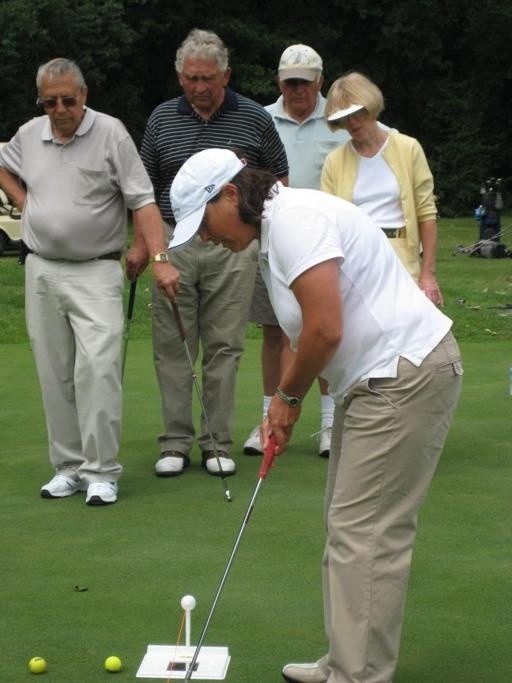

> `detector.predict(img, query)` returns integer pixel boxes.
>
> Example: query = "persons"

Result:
[168,148,461,682]
[320,70,444,305]
[244,41,397,456]
[1,59,181,505]
[126,28,289,475]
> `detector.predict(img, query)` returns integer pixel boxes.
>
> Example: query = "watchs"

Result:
[275,387,303,405]
[153,252,170,263]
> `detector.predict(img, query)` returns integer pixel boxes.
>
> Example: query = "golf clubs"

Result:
[166,294,232,505]
[485,177,502,192]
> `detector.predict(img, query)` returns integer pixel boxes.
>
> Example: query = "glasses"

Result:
[36,92,82,107]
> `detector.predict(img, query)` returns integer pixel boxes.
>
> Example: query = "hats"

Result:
[166,147,245,249]
[327,103,364,121]
[278,43,323,81]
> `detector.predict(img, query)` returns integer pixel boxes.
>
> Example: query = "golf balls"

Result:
[29,656,46,673]
[104,657,122,670]
[181,595,196,611]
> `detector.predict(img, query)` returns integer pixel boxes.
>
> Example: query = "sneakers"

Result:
[202,449,235,475]
[85,482,117,504]
[40,473,88,497]
[155,450,189,475]
[282,662,328,681]
[243,425,264,454]
[319,426,332,455]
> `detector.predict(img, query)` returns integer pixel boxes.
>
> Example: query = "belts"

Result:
[90,250,121,260]
[382,228,406,238]
